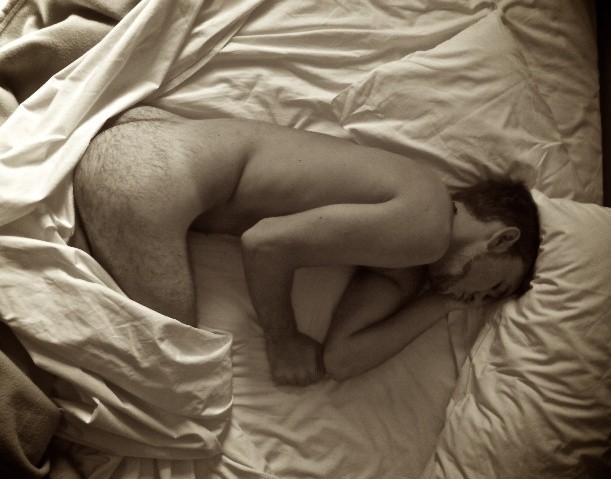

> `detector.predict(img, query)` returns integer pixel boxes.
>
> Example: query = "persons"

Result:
[71,107,540,386]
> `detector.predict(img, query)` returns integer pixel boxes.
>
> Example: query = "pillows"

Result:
[420,188,611,479]
[327,9,590,204]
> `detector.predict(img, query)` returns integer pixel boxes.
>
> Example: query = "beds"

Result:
[1,0,610,478]
